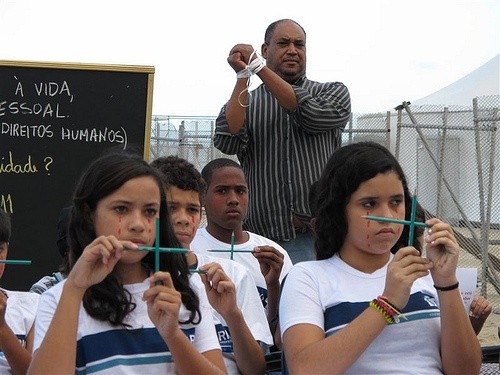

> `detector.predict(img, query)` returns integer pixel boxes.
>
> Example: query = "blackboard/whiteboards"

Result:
[0,61,155,293]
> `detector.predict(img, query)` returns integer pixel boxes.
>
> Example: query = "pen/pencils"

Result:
[0,260,32,265]
[207,231,259,260]
[361,189,432,247]
[123,217,194,286]
[188,269,210,274]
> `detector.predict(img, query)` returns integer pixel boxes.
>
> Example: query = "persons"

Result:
[214,19,351,265]
[0,206,76,375]
[190,158,293,375]
[25,152,229,375]
[148,156,276,375]
[279,140,493,375]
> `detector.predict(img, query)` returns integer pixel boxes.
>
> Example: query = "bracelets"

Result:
[433,281,459,291]
[369,295,408,324]
